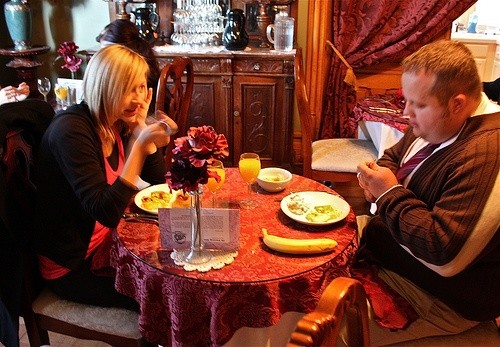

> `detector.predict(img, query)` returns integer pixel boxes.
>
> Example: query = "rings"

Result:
[11,93,13,97]
[357,173,360,177]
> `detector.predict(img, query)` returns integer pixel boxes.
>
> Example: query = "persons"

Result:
[0,54,30,106]
[96,19,171,116]
[29,43,179,313]
[351,40,500,347]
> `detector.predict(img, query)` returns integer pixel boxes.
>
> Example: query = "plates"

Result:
[279,191,350,225]
[134,184,184,215]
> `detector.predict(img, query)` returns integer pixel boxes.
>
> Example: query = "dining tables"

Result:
[105,169,361,347]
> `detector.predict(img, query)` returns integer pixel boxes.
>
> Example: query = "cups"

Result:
[266,17,294,54]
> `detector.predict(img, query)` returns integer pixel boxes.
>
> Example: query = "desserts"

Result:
[140,191,193,211]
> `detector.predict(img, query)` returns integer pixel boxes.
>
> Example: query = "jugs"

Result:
[131,8,160,46]
[222,8,250,51]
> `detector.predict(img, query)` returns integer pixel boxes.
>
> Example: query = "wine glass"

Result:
[54,86,69,111]
[170,0,226,48]
[37,77,51,102]
[205,160,225,208]
[239,153,261,210]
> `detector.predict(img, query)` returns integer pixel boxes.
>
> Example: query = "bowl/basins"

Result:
[256,167,293,192]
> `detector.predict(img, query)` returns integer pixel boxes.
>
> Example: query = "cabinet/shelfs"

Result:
[355,21,497,101]
[153,57,294,173]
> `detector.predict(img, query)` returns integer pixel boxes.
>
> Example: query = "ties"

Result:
[394,142,441,185]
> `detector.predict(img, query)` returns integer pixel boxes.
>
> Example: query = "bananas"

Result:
[262,228,338,254]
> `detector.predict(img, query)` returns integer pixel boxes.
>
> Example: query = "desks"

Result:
[342,86,500,158]
[0,45,50,98]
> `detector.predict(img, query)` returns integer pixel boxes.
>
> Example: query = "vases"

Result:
[4,0,32,51]
[184,185,211,265]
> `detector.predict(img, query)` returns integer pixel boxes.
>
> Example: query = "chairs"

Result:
[294,48,379,183]
[285,277,366,347]
[155,56,195,181]
[0,100,156,347]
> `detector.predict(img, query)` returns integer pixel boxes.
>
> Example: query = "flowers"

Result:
[164,122,229,259]
[54,41,83,79]
